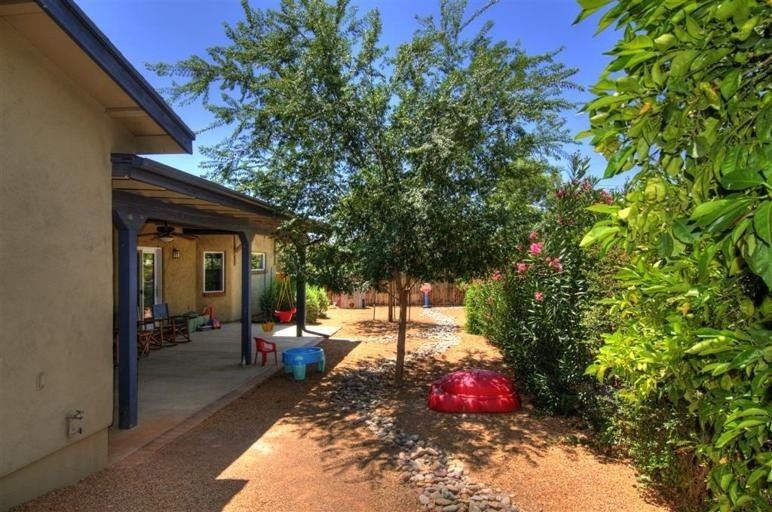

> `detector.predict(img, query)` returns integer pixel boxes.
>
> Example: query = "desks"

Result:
[283,346,325,379]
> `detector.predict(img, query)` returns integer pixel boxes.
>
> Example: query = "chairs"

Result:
[137,303,192,358]
[254,337,277,366]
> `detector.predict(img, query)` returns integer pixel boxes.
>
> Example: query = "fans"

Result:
[136,222,199,242]
